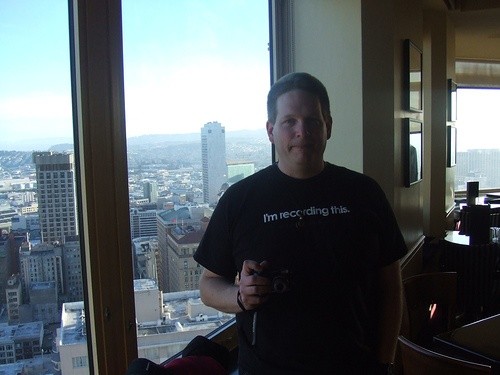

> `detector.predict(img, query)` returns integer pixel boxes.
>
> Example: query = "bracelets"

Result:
[236,288,247,312]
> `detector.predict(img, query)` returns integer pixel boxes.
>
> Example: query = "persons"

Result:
[193,73,409,375]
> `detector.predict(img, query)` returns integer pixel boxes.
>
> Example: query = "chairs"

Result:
[397,335,492,375]
[402,272,457,336]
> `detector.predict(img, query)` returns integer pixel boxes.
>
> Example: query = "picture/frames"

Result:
[402,38,424,111]
[447,78,458,120]
[447,125,457,167]
[403,116,423,187]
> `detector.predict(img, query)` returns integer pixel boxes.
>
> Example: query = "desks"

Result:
[439,200,500,313]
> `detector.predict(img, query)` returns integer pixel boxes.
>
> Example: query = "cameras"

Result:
[250,261,289,318]
